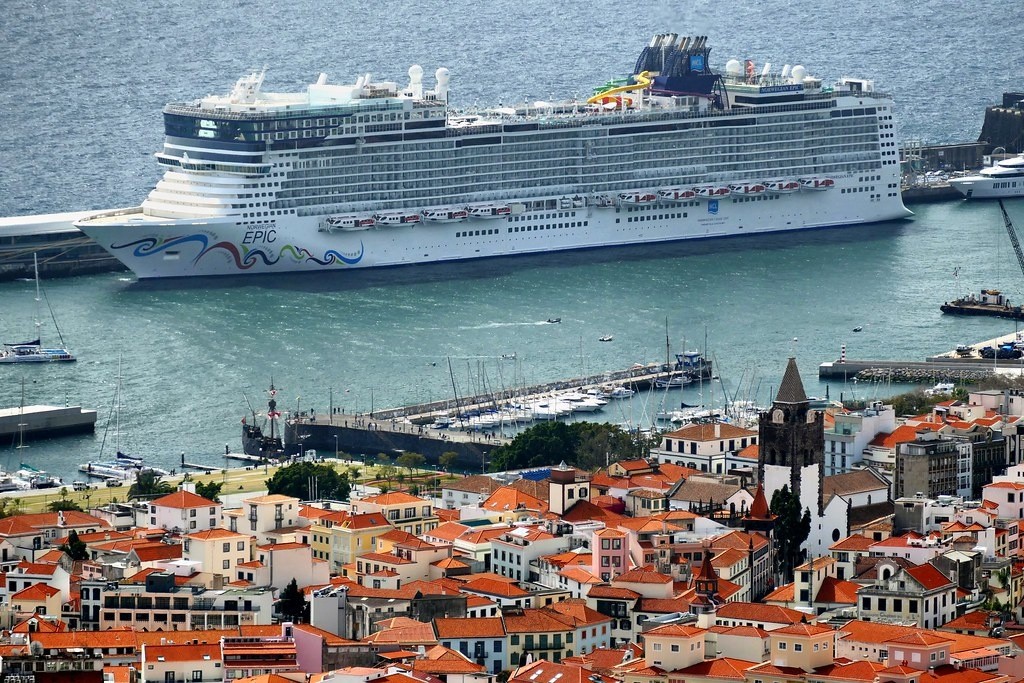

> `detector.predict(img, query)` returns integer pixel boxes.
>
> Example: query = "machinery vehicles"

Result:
[994,196,1024,290]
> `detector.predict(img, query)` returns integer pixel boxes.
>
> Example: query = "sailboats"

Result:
[0,316,773,491]
[0,252,77,363]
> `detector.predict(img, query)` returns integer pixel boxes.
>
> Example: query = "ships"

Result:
[72,33,917,281]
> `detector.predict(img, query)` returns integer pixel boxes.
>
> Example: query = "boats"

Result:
[693,187,731,200]
[654,189,696,202]
[798,177,836,192]
[762,180,800,193]
[421,206,469,226]
[371,210,421,228]
[616,192,658,205]
[947,151,1024,199]
[328,213,375,233]
[462,204,512,219]
[940,289,1024,321]
[729,184,767,197]
